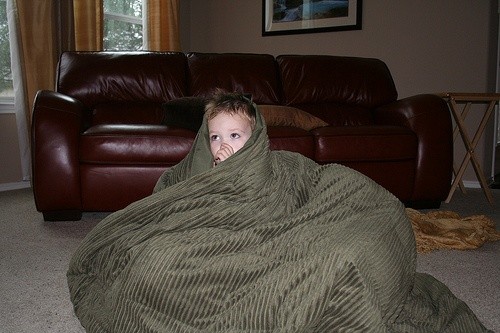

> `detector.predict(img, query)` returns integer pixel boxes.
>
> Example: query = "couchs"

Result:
[30,51,453,221]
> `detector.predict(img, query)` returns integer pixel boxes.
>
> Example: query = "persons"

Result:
[149,85,271,198]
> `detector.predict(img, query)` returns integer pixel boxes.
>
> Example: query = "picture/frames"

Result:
[261,0,361,37]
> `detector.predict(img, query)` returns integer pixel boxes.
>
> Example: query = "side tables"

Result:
[433,92,500,204]
